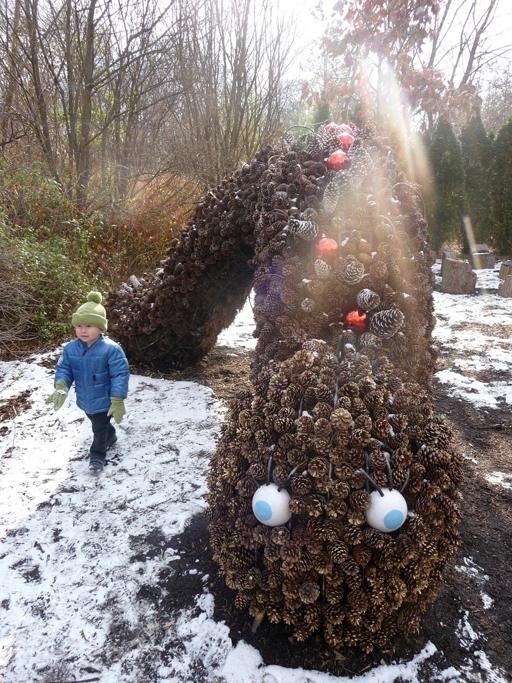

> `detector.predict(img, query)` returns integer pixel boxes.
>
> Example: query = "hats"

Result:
[71,291,107,331]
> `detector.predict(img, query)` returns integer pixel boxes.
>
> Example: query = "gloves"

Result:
[107,397,126,424]
[45,379,68,411]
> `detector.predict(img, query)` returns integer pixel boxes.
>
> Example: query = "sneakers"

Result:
[88,434,117,475]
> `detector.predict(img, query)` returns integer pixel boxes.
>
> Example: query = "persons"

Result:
[53,325,130,476]
